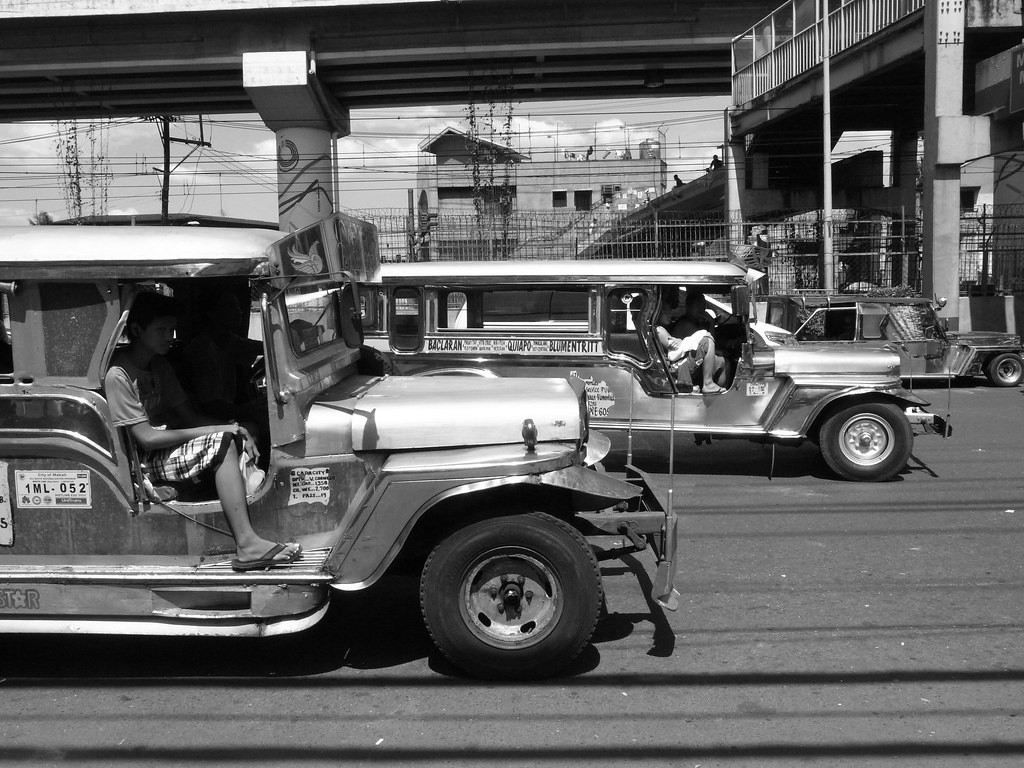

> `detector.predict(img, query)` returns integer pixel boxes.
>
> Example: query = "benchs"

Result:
[116,331,195,501]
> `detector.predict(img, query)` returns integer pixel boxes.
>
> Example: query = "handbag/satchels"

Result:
[238,451,265,496]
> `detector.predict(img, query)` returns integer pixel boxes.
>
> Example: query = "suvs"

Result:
[0,226,681,683]
[345,255,953,483]
[762,291,1023,387]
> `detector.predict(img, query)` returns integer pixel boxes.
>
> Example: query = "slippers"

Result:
[232,542,302,568]
[702,387,726,395]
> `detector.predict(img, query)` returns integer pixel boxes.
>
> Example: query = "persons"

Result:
[586,146,593,160]
[102,291,302,569]
[182,290,270,463]
[674,175,683,188]
[710,156,722,171]
[654,319,726,392]
[669,289,723,355]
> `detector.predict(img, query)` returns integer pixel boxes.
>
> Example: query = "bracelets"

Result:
[233,422,240,436]
